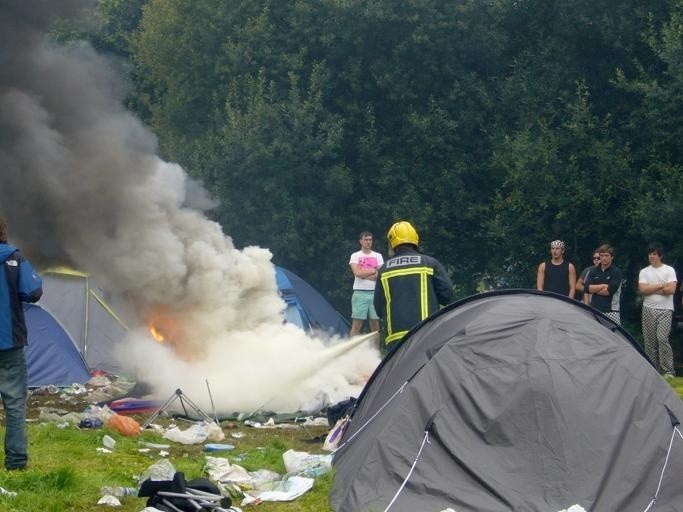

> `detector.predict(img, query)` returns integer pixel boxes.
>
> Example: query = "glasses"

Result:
[592,256,600,260]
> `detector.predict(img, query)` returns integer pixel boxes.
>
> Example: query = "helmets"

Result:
[385,220,420,250]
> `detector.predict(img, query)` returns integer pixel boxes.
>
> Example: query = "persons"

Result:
[0,216,45,473]
[576,247,602,306]
[348,231,380,338]
[639,242,679,377]
[584,244,624,326]
[372,220,454,354]
[536,239,577,300]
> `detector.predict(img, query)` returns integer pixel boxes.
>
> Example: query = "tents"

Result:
[31,265,138,376]
[274,267,352,336]
[15,295,93,390]
[329,288,682,512]
